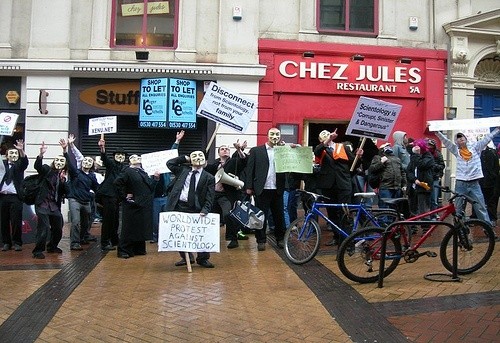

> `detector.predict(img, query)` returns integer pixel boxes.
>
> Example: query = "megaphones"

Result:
[215,168,245,189]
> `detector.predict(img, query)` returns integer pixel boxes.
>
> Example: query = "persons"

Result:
[0,139,29,251]
[314,128,364,257]
[59,130,250,259]
[0,142,8,155]
[266,127,500,246]
[246,127,297,251]
[32,141,70,259]
[426,122,500,250]
[166,150,216,268]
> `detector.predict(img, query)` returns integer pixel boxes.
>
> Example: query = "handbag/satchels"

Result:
[18,174,49,205]
[368,155,382,189]
[230,193,265,230]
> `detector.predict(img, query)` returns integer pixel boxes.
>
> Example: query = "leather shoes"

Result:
[33,235,146,259]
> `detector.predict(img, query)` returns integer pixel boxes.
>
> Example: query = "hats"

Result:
[427,139,436,146]
[113,147,128,158]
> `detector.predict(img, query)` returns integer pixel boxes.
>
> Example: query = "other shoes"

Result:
[227,240,239,249]
[290,226,298,232]
[421,228,431,237]
[258,242,265,251]
[238,235,249,240]
[435,213,440,219]
[354,239,365,247]
[175,258,195,266]
[490,220,496,226]
[324,237,336,246]
[483,228,496,237]
[308,227,316,235]
[196,259,215,268]
[15,244,21,250]
[1,244,10,250]
[327,224,332,230]
[410,226,418,234]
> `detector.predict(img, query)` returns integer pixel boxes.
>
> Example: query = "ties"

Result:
[188,170,199,211]
[55,173,60,202]
[5,163,15,185]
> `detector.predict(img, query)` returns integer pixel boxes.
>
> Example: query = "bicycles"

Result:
[284,190,411,265]
[337,185,495,284]
